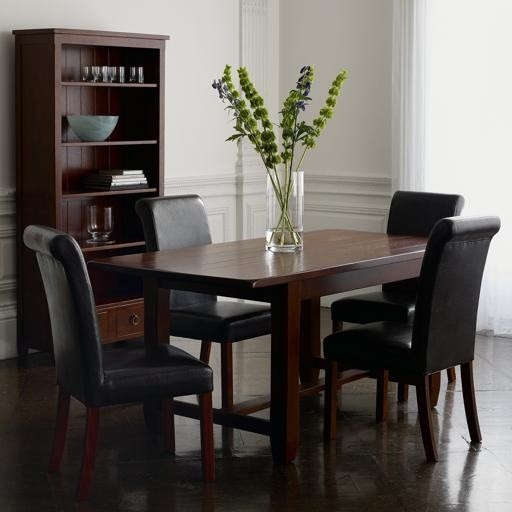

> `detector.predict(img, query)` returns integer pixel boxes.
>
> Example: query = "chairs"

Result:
[135,192,270,446]
[23,223,218,507]
[326,189,470,411]
[318,212,501,501]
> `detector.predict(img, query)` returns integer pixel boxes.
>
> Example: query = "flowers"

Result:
[211,62,350,246]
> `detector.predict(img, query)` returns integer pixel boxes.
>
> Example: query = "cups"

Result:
[80,65,145,84]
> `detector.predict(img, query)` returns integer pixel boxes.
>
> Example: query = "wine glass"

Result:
[86,206,115,244]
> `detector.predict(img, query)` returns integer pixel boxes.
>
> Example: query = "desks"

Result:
[83,227,432,468]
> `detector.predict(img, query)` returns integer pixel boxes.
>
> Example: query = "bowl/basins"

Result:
[67,115,120,141]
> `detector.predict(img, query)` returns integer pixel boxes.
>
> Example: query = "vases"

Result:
[266,170,303,253]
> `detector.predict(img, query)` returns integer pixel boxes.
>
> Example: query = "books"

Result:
[83,169,149,190]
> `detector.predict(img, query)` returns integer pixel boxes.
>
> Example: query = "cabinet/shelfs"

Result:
[11,28,171,357]
